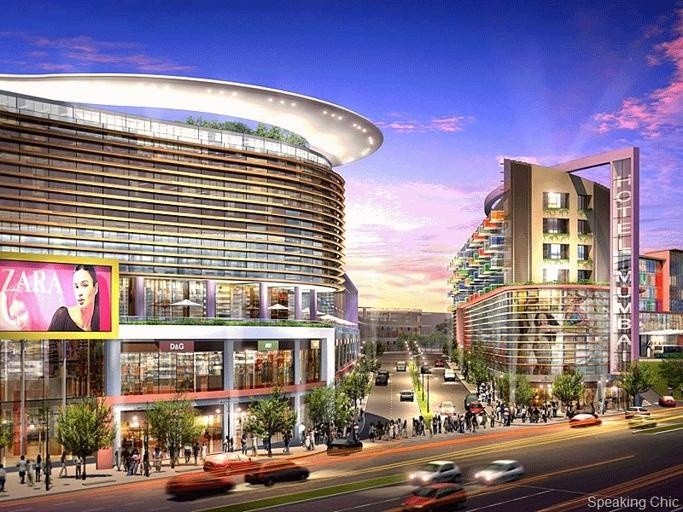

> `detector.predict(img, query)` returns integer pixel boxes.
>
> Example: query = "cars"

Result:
[655,395,677,407]
[377,369,390,377]
[437,401,456,416]
[242,460,310,488]
[398,390,415,402]
[205,453,259,476]
[434,358,444,367]
[568,413,602,427]
[325,437,363,457]
[402,483,467,512]
[627,415,656,428]
[373,375,387,386]
[164,474,238,499]
[408,460,463,487]
[474,459,523,485]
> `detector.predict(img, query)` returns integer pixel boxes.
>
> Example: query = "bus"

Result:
[652,344,682,359]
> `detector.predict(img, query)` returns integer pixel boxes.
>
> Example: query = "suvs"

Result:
[469,402,486,415]
[463,394,478,410]
[624,406,650,419]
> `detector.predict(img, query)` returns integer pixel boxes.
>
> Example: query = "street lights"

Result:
[621,377,633,410]
[576,380,583,404]
[350,336,366,410]
[404,339,431,413]
[132,404,161,478]
[27,413,69,492]
[538,376,552,417]
[498,374,503,399]
[216,400,242,454]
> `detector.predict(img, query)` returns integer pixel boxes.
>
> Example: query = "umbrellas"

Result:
[303,306,325,315]
[168,299,203,317]
[267,303,290,319]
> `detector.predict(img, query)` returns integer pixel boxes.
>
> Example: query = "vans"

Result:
[442,369,457,382]
[394,360,406,372]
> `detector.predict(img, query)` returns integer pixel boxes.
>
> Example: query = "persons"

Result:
[115,441,208,477]
[517,313,560,375]
[17,449,82,484]
[369,383,608,443]
[0,464,6,492]
[221,421,359,455]
[0,264,100,332]
